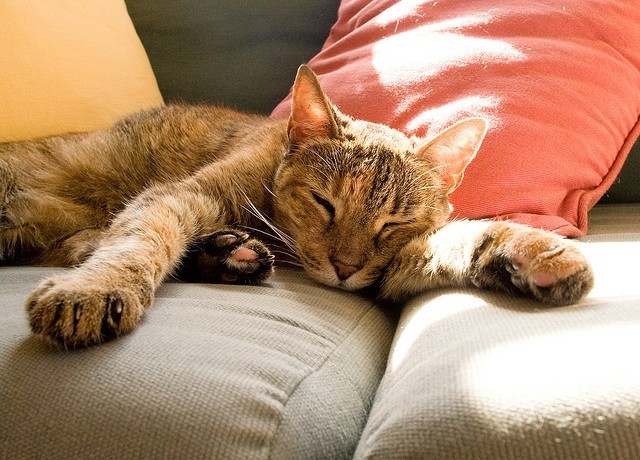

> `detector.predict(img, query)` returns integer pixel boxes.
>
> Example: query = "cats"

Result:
[0,63,597,350]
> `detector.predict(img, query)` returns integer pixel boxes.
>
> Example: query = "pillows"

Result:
[0,0,165,142]
[269,0,640,243]
